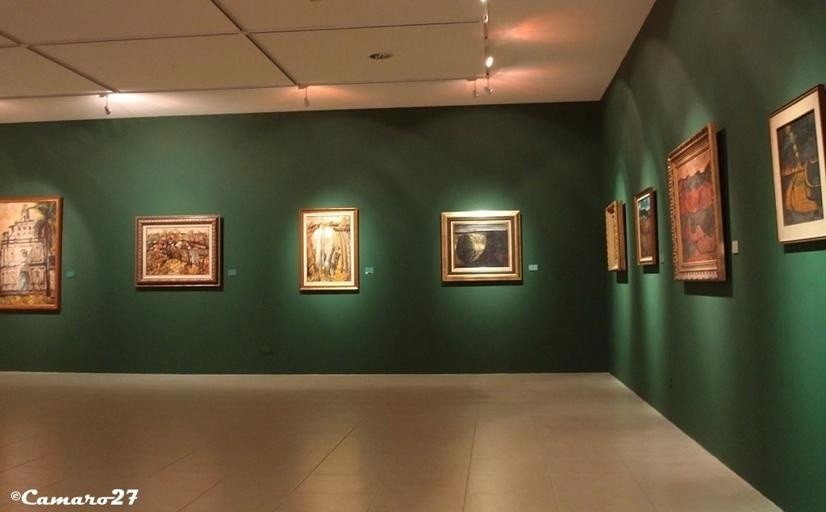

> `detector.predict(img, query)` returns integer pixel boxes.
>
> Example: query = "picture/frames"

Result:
[135,215,222,288]
[0,196,62,312]
[666,123,726,282]
[604,200,626,272]
[632,186,657,266]
[440,209,522,282]
[298,207,359,294]
[767,83,826,245]
